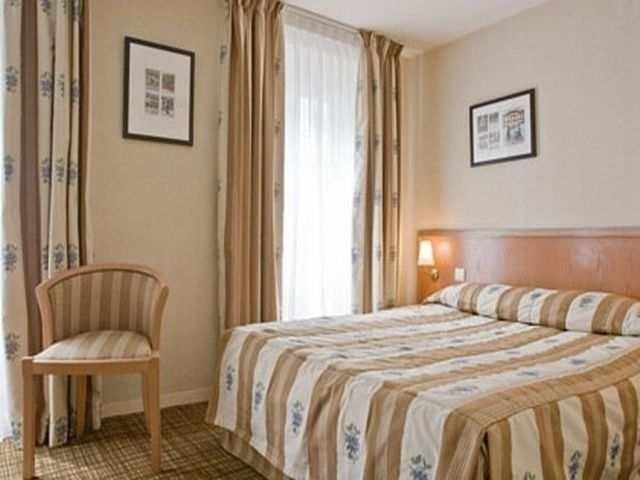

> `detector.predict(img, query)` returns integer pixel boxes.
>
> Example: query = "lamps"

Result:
[416,239,440,282]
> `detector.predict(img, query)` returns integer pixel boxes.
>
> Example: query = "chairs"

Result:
[19,257,169,480]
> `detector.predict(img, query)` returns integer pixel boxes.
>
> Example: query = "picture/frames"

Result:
[468,88,538,169]
[120,34,197,149]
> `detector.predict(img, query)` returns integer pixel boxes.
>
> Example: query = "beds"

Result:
[201,277,640,480]
